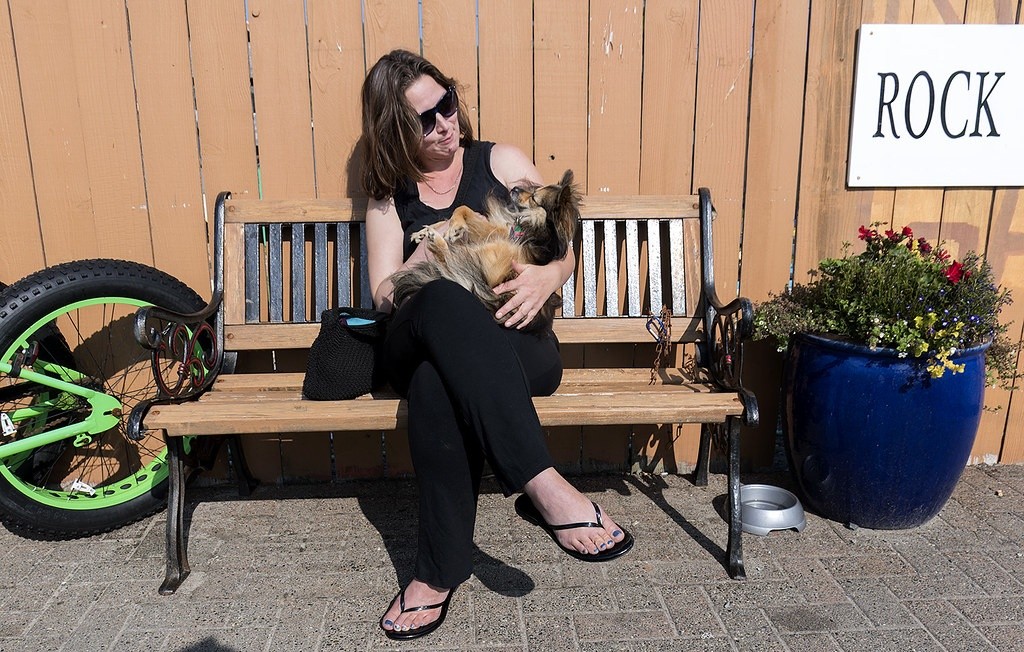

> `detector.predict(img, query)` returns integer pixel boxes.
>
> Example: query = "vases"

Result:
[784,325,992,530]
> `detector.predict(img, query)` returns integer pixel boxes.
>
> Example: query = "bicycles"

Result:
[0,260,236,538]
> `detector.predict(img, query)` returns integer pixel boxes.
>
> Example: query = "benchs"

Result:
[129,187,760,597]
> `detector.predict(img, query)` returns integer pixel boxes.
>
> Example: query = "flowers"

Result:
[742,215,1024,399]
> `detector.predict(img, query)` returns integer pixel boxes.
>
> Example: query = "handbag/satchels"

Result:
[301,306,393,401]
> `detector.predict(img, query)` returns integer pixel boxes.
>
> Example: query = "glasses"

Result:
[417,84,460,136]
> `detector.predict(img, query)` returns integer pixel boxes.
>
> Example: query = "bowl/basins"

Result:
[722,485,806,537]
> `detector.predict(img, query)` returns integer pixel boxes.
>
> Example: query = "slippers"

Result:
[381,577,471,640]
[515,492,632,562]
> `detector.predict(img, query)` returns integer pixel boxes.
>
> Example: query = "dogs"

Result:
[391,169,585,328]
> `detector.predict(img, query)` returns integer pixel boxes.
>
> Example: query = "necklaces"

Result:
[422,164,463,194]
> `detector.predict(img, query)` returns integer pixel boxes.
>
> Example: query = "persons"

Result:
[362,48,634,640]
